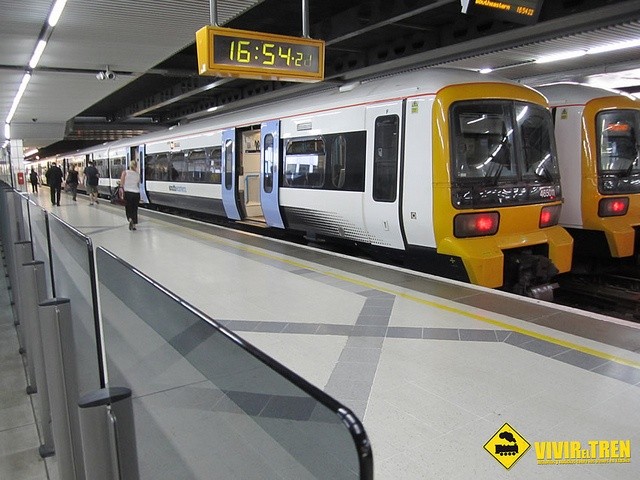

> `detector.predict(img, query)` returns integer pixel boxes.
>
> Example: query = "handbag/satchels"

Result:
[111,187,124,201]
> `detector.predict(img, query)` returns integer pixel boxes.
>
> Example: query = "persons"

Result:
[30,169,38,193]
[81,161,100,205]
[45,162,63,206]
[117,160,141,231]
[66,165,81,201]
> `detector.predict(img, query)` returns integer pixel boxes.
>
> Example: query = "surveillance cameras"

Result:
[32,117,37,122]
[96,64,116,80]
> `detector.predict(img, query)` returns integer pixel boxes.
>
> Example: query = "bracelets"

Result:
[120,187,123,188]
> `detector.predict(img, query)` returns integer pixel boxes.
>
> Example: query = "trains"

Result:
[25,66,574,303]
[537,83,640,260]
